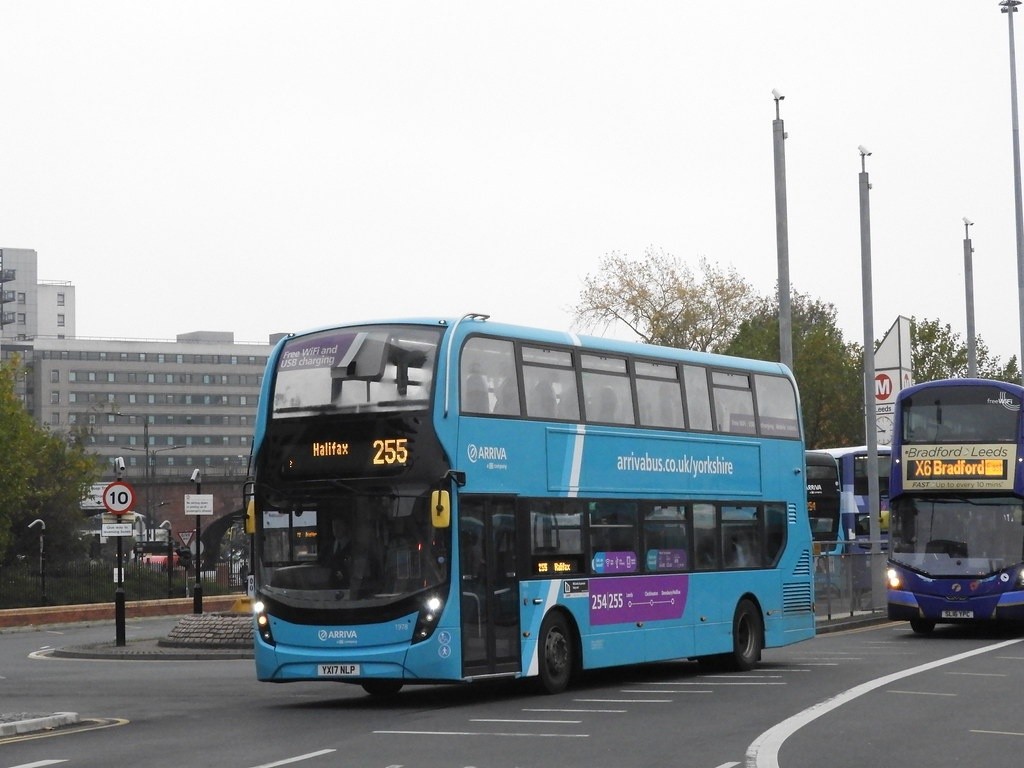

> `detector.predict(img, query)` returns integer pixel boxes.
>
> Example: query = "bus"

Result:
[887,378,1024,634]
[243,313,817,694]
[805,444,892,554]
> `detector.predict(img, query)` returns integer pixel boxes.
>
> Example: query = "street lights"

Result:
[106,407,187,540]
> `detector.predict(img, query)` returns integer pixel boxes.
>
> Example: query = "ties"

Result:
[335,540,341,554]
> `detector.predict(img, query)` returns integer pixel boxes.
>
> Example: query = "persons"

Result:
[726,535,745,566]
[906,519,937,544]
[301,517,358,576]
